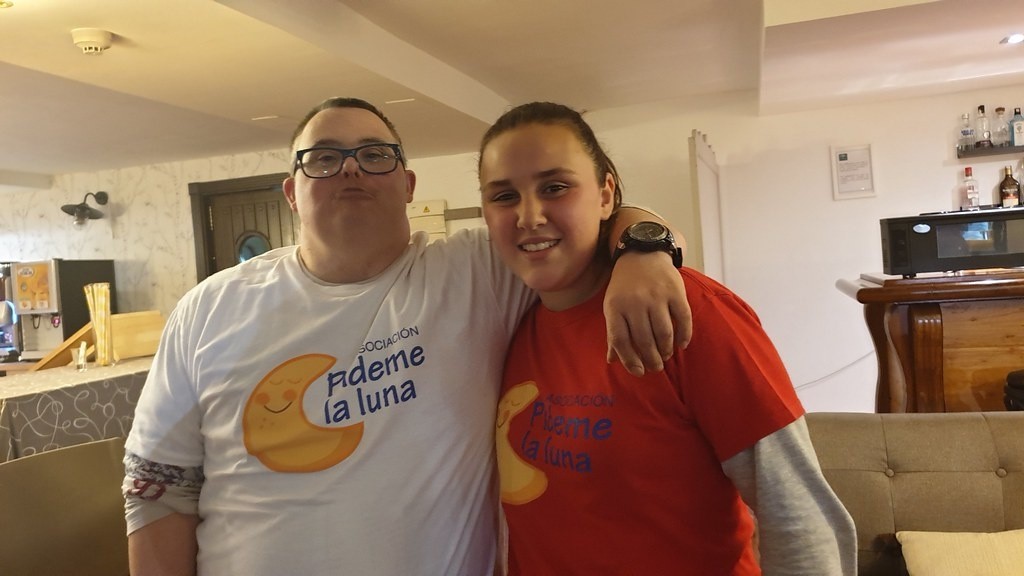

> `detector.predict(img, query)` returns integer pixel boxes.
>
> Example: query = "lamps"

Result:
[61,192,108,231]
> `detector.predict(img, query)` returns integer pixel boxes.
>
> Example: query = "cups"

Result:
[70,347,87,373]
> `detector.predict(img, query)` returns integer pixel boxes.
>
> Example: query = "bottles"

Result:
[1000,165,1021,208]
[956,113,976,157]
[976,105,991,150]
[1008,108,1024,148]
[959,167,980,212]
[990,107,1011,148]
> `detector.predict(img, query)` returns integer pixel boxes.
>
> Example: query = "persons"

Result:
[121,94,693,576]
[479,102,860,576]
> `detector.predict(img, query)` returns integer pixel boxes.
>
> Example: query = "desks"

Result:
[835,271,1024,411]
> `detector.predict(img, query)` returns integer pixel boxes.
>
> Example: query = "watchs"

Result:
[613,220,685,268]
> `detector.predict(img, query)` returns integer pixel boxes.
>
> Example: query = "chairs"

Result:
[0,437,129,576]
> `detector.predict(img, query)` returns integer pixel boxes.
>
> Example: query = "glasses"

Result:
[288,144,406,179]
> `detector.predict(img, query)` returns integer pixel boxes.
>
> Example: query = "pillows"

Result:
[896,529,1024,576]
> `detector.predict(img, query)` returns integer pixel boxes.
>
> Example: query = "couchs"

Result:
[803,411,1024,576]
